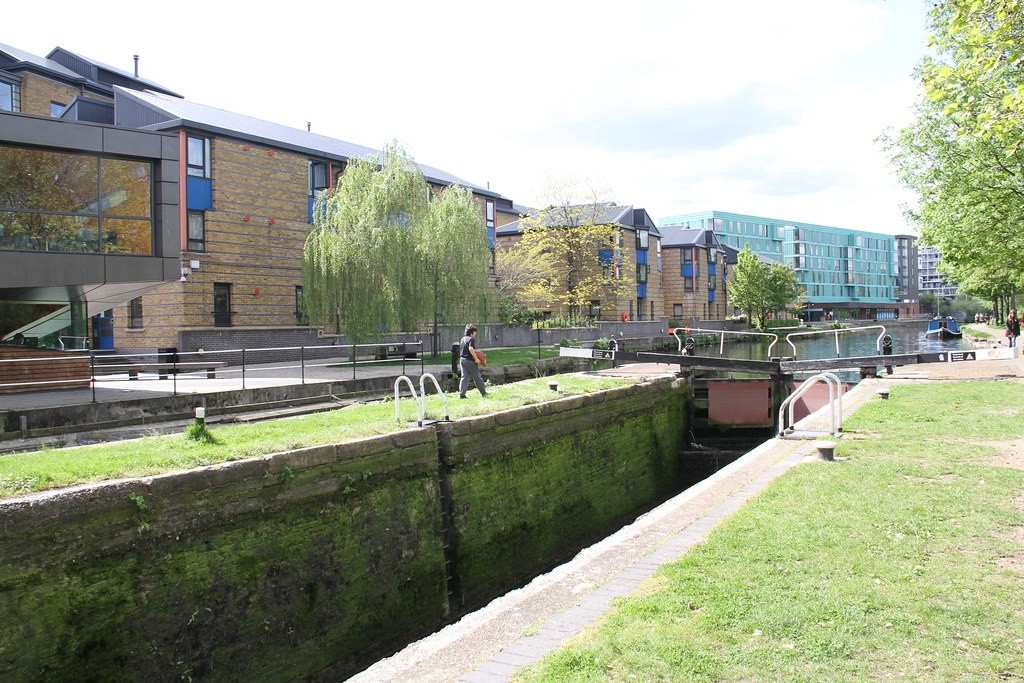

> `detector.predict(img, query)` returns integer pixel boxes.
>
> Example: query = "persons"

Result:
[1006,308,1020,347]
[13,333,24,345]
[459,323,491,398]
[826,313,832,320]
[895,313,898,319]
[975,312,989,325]
[1021,314,1024,355]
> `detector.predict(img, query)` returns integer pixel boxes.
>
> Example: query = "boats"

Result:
[926,290,963,342]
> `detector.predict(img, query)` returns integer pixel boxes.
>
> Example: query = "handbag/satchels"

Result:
[1006,331,1013,337]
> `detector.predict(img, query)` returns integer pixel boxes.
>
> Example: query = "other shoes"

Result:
[486,392,491,395]
[460,396,468,399]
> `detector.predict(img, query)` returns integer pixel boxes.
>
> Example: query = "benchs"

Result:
[368,350,422,362]
[89,361,227,381]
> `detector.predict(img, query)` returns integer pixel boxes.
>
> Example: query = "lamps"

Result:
[196,406,205,418]
[548,381,559,386]
